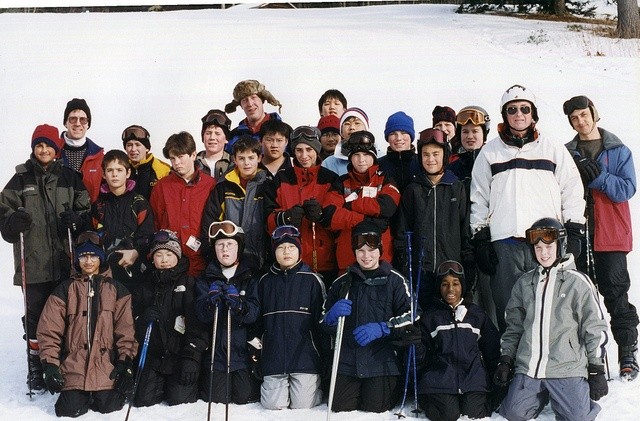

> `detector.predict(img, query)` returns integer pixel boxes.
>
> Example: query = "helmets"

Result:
[211,220,244,260]
[418,130,452,170]
[529,219,568,262]
[455,106,489,141]
[500,85,539,122]
[348,131,377,164]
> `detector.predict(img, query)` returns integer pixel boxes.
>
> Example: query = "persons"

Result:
[19,124,91,376]
[432,105,460,164]
[376,111,419,188]
[87,149,153,277]
[448,107,490,198]
[224,80,293,155]
[394,130,471,315]
[321,130,401,277]
[58,98,105,204]
[135,230,208,405]
[563,93,637,381]
[321,108,370,176]
[34,229,138,416]
[319,221,421,414]
[195,221,260,405]
[194,109,237,182]
[402,261,500,421]
[150,132,217,277]
[246,225,327,409]
[259,119,294,177]
[36,241,139,377]
[263,126,340,283]
[498,219,609,421]
[121,125,171,198]
[318,116,342,161]
[200,135,268,268]
[319,88,348,119]
[470,84,587,330]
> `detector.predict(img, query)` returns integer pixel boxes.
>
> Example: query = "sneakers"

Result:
[619,346,638,379]
[26,354,42,391]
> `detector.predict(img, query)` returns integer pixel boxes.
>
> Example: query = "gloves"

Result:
[6,211,32,237]
[179,349,200,386]
[42,363,62,395]
[304,200,325,221]
[575,155,600,183]
[474,228,498,275]
[138,307,160,335]
[352,322,391,347]
[588,365,607,401]
[250,355,263,381]
[403,321,422,349]
[324,299,352,326]
[564,223,585,261]
[209,281,224,309]
[496,355,513,386]
[110,355,136,396]
[58,210,83,237]
[276,205,304,228]
[225,284,242,314]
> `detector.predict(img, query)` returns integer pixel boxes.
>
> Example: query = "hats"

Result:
[271,228,301,256]
[433,106,455,129]
[437,260,464,296]
[123,126,150,149]
[75,234,105,267]
[339,108,368,137]
[31,125,64,159]
[64,99,91,129]
[226,80,281,114]
[147,228,182,262]
[202,110,231,139]
[384,113,415,141]
[352,223,382,256]
[317,115,339,133]
[290,127,322,154]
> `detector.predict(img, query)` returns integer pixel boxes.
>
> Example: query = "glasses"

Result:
[271,225,301,240]
[563,97,593,115]
[75,232,103,246]
[123,126,148,139]
[351,231,382,249]
[526,228,567,244]
[456,109,489,126]
[143,232,181,253]
[349,134,373,144]
[67,116,89,125]
[505,106,532,114]
[436,260,464,276]
[208,220,244,239]
[421,129,447,144]
[203,112,229,125]
[290,126,320,141]
